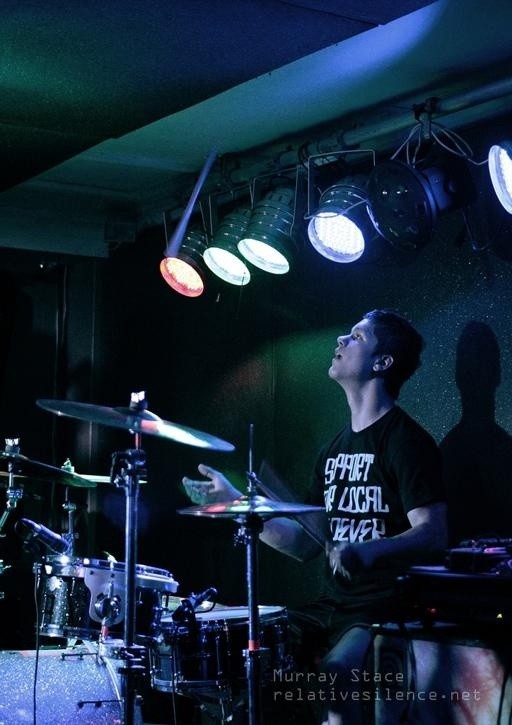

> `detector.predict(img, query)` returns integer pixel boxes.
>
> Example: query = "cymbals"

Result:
[74,472,150,489]
[1,451,97,489]
[36,398,234,453]
[179,496,327,518]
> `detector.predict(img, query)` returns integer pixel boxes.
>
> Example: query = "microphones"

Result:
[20,517,70,555]
[171,588,214,622]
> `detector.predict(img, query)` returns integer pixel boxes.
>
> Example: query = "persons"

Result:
[180,307,451,725]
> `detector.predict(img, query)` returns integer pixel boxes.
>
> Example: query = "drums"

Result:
[151,604,291,693]
[157,592,226,624]
[0,639,145,725]
[35,556,179,645]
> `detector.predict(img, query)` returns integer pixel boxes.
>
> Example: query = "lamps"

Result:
[160,77,512,297]
